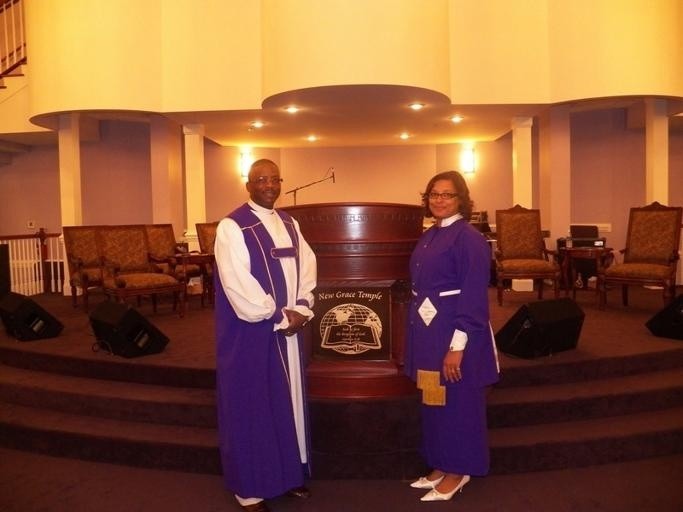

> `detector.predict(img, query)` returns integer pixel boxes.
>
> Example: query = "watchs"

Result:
[298,315,307,330]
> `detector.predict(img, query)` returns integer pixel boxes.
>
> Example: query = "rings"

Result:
[288,332,290,333]
[456,368,461,371]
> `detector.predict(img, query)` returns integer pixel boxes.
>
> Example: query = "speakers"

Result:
[643,292,683,339]
[0,291,64,340]
[493,296,585,357]
[88,296,169,357]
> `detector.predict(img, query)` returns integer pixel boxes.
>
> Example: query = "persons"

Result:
[213,159,318,512]
[403,171,501,502]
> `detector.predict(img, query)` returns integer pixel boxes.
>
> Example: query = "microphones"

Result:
[331,166,336,184]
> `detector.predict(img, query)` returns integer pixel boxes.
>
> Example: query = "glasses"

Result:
[428,193,458,200]
[249,178,282,184]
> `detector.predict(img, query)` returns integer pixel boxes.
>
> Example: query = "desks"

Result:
[559,245,614,304]
[169,253,214,311]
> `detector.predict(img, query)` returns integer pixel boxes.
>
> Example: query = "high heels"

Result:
[411,473,445,490]
[421,475,471,502]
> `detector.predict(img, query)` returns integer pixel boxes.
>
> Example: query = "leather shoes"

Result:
[283,488,311,501]
[230,493,270,512]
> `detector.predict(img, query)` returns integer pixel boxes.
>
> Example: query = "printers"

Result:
[566,224,604,247]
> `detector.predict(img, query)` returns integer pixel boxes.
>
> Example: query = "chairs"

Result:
[596,201,683,312]
[196,221,220,309]
[494,204,561,307]
[62,223,184,315]
